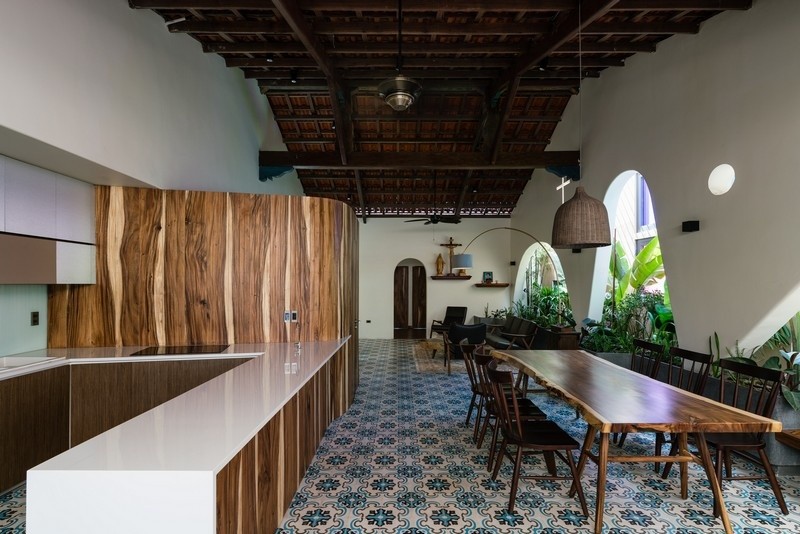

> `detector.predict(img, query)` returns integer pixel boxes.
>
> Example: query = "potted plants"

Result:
[495,235,800,476]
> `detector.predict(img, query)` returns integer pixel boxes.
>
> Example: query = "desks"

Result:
[492,350,783,534]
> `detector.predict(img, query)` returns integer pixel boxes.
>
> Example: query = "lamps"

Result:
[540,59,549,71]
[290,71,297,84]
[377,0,423,111]
[452,227,572,332]
[265,53,275,63]
[552,0,610,249]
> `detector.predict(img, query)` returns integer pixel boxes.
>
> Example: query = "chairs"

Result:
[430,306,790,516]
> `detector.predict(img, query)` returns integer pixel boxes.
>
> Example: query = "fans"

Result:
[404,169,461,225]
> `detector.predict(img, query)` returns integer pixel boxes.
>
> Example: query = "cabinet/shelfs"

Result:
[0,358,254,496]
[0,153,97,284]
